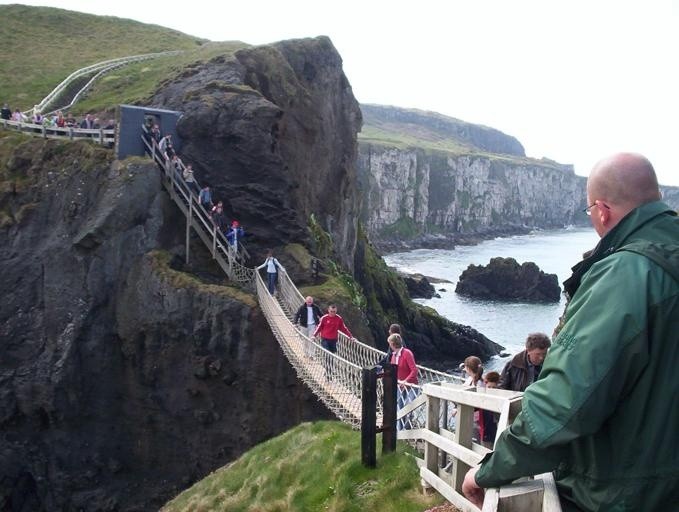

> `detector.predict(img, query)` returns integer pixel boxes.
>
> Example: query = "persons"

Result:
[256,253,286,297]
[310,305,357,382]
[150,124,245,267]
[293,296,325,362]
[375,324,419,431]
[1,103,116,147]
[464,332,550,448]
[461,151,679,512]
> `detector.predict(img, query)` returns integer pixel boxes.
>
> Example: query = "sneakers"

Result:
[302,351,314,360]
[322,372,333,382]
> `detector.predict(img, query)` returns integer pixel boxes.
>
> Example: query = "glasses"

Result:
[583,199,610,217]
